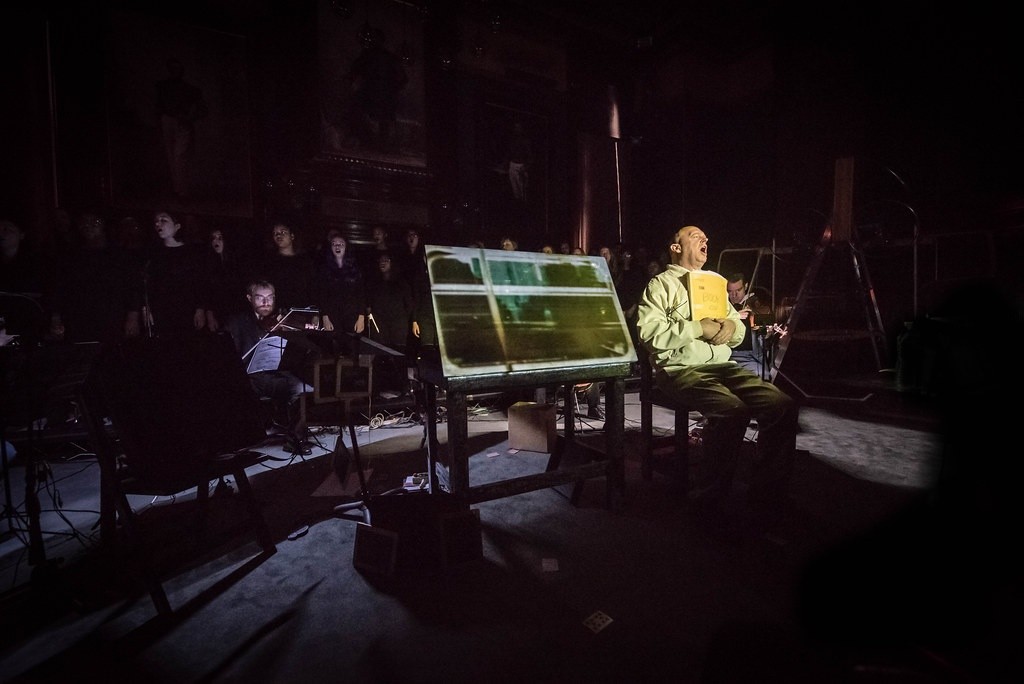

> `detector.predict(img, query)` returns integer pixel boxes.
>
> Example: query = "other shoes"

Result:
[588,405,607,422]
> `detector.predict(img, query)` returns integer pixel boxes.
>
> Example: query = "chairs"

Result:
[633,302,699,508]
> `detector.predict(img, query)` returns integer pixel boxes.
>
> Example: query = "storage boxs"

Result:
[508,402,557,453]
[299,358,372,405]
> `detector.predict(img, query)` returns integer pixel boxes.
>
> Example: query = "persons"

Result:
[635,225,800,495]
[0,208,764,455]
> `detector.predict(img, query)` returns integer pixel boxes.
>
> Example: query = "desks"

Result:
[417,359,631,509]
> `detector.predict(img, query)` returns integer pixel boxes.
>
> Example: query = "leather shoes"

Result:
[282,442,312,455]
